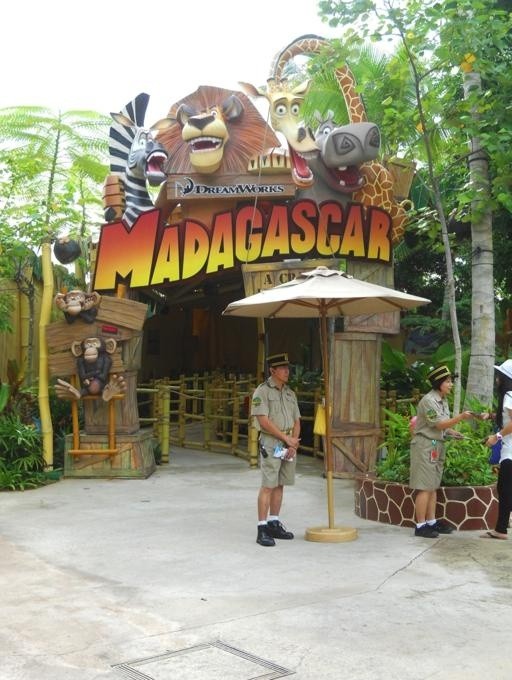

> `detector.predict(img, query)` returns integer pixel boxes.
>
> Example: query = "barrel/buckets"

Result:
[479,530,509,540]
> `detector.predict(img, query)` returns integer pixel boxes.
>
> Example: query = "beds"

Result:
[222,265,432,528]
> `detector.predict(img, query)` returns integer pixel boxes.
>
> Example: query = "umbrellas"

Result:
[256,524,276,547]
[266,519,295,541]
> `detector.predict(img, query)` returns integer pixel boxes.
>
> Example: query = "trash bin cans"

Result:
[496,432,502,441]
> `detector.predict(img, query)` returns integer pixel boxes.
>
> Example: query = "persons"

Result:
[474,359,512,539]
[408,365,474,538]
[251,353,302,546]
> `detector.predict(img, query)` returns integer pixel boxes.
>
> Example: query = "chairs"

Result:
[416,522,440,538]
[427,517,459,536]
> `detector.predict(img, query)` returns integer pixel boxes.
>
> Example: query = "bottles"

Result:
[265,351,291,369]
[490,357,512,381]
[427,365,459,389]
